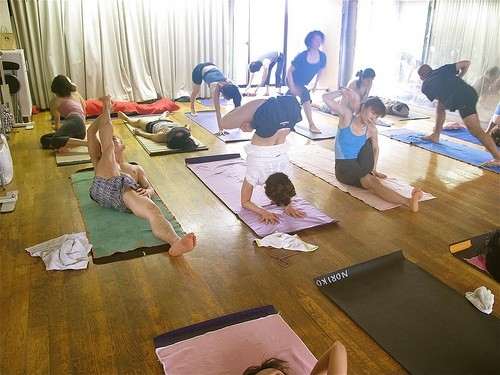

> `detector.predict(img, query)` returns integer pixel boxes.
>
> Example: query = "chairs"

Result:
[0,61,24,123]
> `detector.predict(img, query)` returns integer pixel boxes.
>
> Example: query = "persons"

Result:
[87,96,197,257]
[220,95,306,225]
[40,74,88,154]
[117,110,199,149]
[485,227,500,283]
[243,341,347,375]
[190,30,500,213]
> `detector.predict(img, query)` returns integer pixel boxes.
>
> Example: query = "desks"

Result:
[0,49,32,122]
